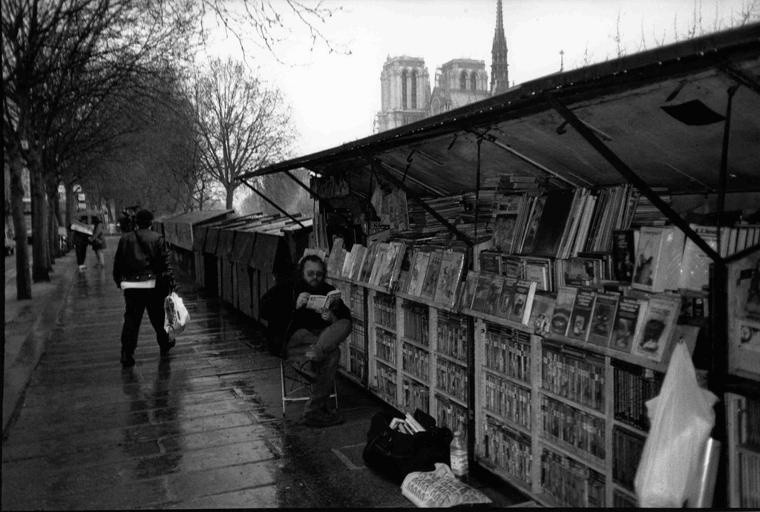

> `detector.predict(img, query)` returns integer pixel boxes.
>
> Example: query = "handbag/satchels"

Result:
[363,410,453,487]
[163,287,190,342]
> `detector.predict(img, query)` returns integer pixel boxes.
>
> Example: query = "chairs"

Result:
[279,358,338,416]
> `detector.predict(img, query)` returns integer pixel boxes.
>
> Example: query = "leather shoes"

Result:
[302,408,345,427]
[159,338,176,357]
[304,345,325,363]
[121,357,135,365]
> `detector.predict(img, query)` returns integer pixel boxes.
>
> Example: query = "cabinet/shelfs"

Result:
[323,275,709,509]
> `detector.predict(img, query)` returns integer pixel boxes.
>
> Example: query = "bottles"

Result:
[449,431,470,486]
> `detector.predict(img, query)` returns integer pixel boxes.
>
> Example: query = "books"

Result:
[298,171,759,510]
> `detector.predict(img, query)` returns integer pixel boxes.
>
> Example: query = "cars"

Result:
[4,224,16,256]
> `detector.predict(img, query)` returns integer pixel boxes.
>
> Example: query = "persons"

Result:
[117,352,185,480]
[68,215,90,270]
[111,209,176,366]
[87,216,107,268]
[257,255,353,427]
[118,211,132,236]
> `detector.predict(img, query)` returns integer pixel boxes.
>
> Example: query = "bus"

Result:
[5,198,31,244]
